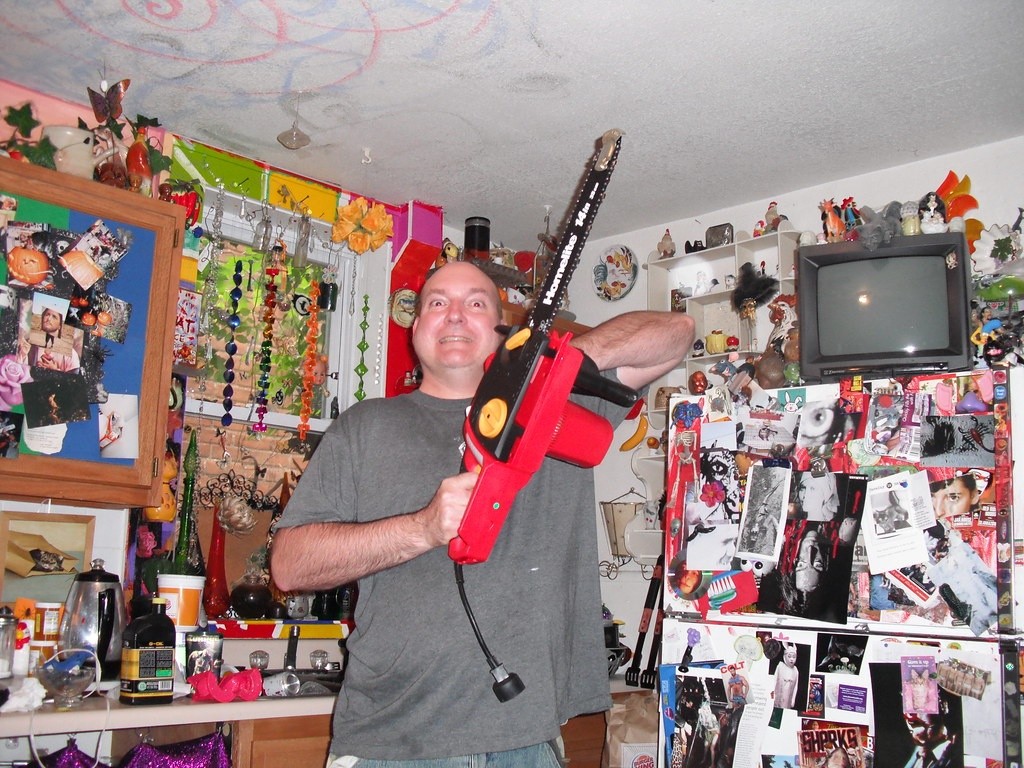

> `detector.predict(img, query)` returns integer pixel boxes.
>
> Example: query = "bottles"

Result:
[120,598,175,706]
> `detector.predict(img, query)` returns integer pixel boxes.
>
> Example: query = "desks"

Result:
[0,670,340,768]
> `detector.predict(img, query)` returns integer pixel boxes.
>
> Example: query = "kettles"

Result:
[56,558,128,680]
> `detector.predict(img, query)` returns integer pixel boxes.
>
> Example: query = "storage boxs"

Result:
[200,621,357,673]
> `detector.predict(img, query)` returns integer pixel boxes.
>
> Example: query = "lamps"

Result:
[600,490,656,582]
[277,94,310,149]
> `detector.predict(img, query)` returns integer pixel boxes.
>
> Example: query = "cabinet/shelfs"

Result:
[624,230,800,565]
[0,155,187,511]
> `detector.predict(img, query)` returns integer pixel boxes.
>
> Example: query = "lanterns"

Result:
[7,246,49,284]
[59,251,104,291]
[71,298,112,337]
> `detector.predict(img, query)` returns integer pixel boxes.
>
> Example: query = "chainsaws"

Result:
[448,127,639,704]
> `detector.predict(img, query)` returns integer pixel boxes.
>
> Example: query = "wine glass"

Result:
[38,667,97,707]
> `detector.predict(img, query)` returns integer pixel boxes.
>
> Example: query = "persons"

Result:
[728,666,750,708]
[900,686,958,768]
[777,519,840,618]
[41,308,63,348]
[902,563,927,583]
[796,472,841,522]
[39,346,80,374]
[929,474,980,517]
[774,641,799,709]
[698,701,721,768]
[799,400,840,447]
[272,261,695,768]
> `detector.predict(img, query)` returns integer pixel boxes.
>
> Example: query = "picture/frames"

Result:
[0,511,96,611]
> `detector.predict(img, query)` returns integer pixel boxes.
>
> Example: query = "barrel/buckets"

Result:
[155,574,206,632]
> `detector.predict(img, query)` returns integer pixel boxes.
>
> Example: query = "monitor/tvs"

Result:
[797,232,975,386]
